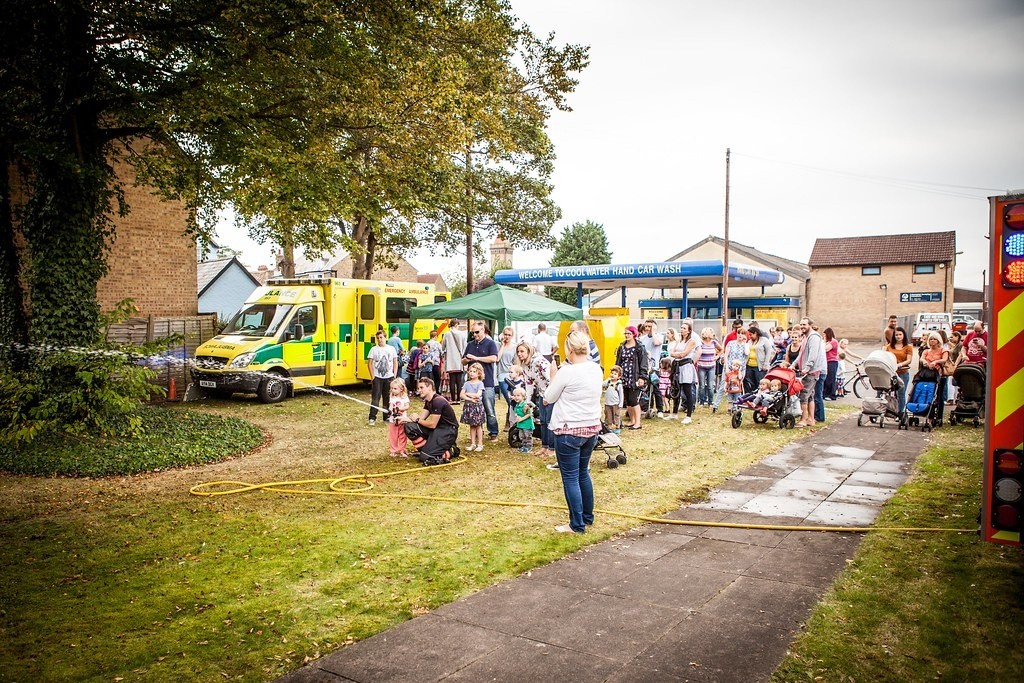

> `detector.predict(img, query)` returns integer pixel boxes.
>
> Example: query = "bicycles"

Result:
[840,361,869,399]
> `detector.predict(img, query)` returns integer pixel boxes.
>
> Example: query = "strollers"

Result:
[503,376,542,448]
[898,363,945,432]
[947,359,986,428]
[592,419,628,468]
[857,350,911,428]
[625,374,657,419]
[726,363,808,431]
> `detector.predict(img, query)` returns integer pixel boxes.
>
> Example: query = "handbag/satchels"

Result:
[402,350,410,362]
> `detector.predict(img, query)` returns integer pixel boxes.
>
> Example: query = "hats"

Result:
[626,326,638,339]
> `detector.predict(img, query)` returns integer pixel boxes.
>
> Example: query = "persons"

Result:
[389,378,410,458]
[637,317,866,428]
[601,365,624,433]
[884,316,988,424]
[379,324,404,379]
[405,320,466,405]
[514,389,534,453]
[485,325,559,431]
[393,378,460,461]
[367,330,398,425]
[543,331,603,533]
[460,363,486,451]
[461,322,499,442]
[616,327,648,430]
[516,342,556,457]
[546,322,601,472]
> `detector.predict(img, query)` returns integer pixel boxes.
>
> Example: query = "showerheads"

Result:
[387,406,400,425]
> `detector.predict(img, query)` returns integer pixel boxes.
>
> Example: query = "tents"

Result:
[409,284,583,352]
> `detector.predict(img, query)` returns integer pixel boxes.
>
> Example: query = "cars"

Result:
[953,313,984,330]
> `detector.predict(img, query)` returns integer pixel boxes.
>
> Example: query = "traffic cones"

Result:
[167,377,179,402]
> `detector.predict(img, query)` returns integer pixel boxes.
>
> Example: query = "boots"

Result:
[503,413,511,432]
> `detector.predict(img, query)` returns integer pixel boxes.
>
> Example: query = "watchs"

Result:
[416,418,420,423]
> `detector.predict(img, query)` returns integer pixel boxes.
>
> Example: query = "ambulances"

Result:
[189,277,453,404]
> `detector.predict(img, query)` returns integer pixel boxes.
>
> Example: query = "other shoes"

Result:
[384,420,389,426]
[465,443,476,451]
[369,419,375,425]
[400,453,407,458]
[407,389,467,406]
[555,524,575,533]
[467,431,485,439]
[519,445,560,471]
[491,435,499,443]
[448,441,460,458]
[605,392,849,436]
[474,444,483,452]
[408,450,421,457]
[389,452,398,458]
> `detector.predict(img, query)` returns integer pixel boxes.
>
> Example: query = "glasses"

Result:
[565,330,576,343]
[734,326,741,329]
[471,330,482,335]
[504,333,512,337]
[624,332,631,336]
